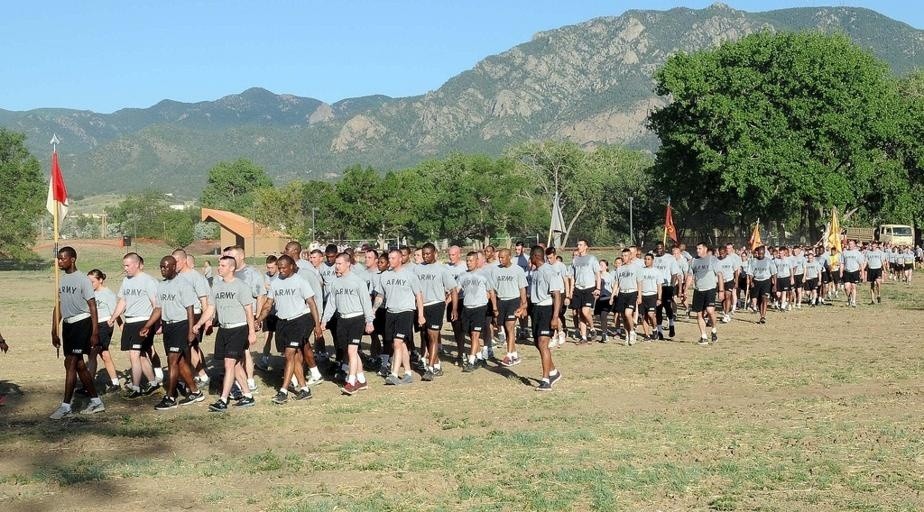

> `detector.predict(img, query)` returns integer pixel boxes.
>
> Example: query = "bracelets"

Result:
[0,339,5,343]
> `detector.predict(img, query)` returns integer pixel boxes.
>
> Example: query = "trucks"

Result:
[845,224,912,249]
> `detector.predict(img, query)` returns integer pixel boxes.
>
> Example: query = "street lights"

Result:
[625,189,634,244]
[311,206,319,244]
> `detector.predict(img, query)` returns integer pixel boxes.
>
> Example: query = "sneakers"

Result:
[49,404,73,419]
[75,388,90,397]
[455,308,692,373]
[80,400,105,415]
[106,340,446,410]
[696,286,883,347]
[536,381,551,391]
[548,370,560,386]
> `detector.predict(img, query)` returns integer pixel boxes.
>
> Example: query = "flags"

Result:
[45,153,69,234]
[665,208,677,242]
[751,226,761,251]
[828,210,842,255]
[552,200,566,241]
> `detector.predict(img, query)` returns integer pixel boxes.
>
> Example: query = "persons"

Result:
[0,334,8,354]
[50,238,330,421]
[307,241,604,391]
[784,240,924,308]
[593,240,822,346]
